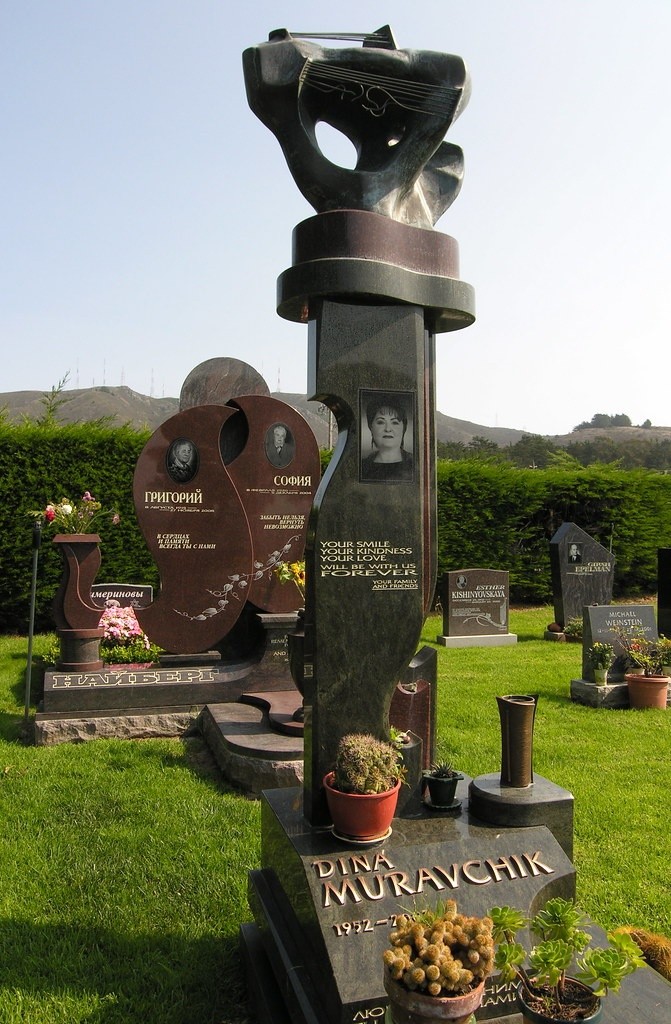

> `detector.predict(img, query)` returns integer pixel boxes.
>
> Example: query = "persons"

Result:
[362,393,412,480]
[266,426,293,468]
[568,544,582,563]
[169,441,194,482]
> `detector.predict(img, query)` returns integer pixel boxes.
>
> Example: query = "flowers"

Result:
[272,559,306,604]
[25,490,121,535]
[628,641,646,674]
[97,599,160,662]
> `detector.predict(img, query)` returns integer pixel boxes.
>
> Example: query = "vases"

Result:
[287,607,306,720]
[629,668,646,675]
[105,662,152,671]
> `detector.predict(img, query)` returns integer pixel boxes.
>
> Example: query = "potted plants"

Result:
[384,877,495,1024]
[609,618,670,709]
[587,642,616,685]
[423,759,464,805]
[563,616,584,643]
[322,732,412,841]
[491,896,646,1024]
